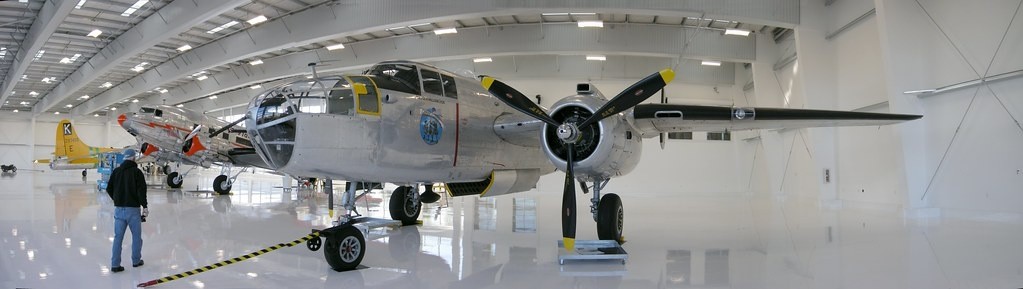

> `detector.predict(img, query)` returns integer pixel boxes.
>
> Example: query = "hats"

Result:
[124,148,137,159]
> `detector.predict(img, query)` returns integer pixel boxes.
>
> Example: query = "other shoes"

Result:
[133,260,144,267]
[111,266,124,272]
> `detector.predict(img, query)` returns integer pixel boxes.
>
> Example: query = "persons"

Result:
[106,149,149,272]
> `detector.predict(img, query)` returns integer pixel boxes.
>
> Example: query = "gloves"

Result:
[142,208,149,217]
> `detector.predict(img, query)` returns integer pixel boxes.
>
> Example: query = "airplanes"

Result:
[238,57,926,266]
[120,105,325,196]
[33,120,174,176]
